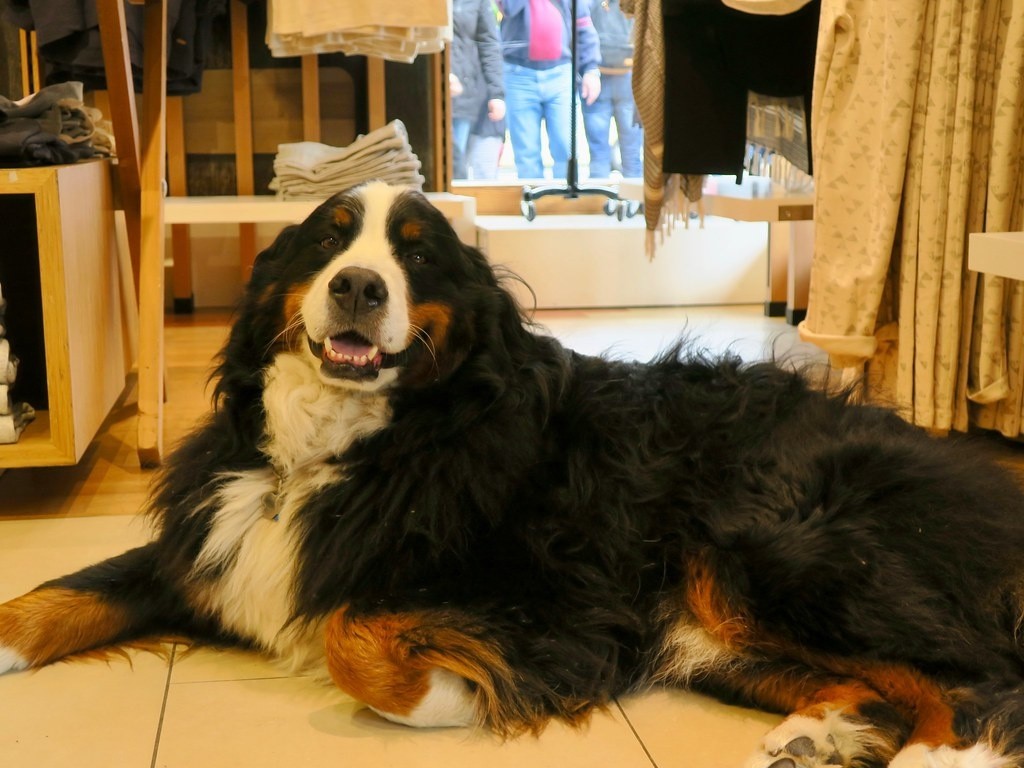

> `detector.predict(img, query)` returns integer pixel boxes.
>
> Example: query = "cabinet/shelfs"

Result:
[0,160,127,465]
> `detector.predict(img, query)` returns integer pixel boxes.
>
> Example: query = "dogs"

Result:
[0,179,1023,768]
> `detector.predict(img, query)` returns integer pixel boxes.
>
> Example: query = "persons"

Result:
[467,102,507,180]
[501,0,602,178]
[449,0,507,179]
[579,2,644,178]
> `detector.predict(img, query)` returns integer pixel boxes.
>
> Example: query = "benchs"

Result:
[161,190,476,250]
